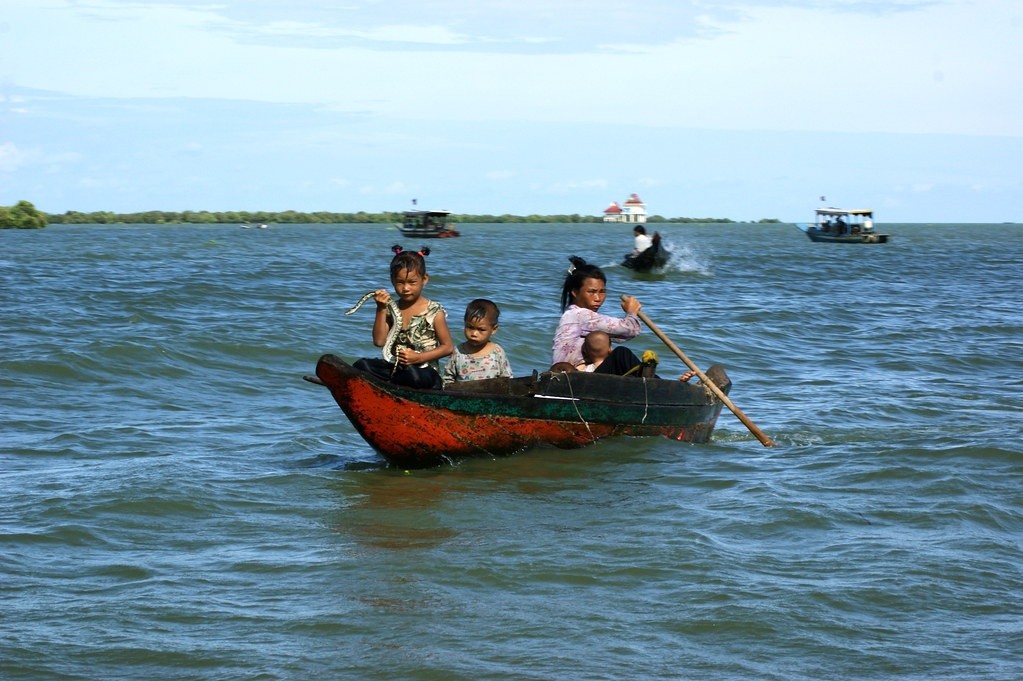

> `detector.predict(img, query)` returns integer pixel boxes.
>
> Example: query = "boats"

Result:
[794,206,890,246]
[391,205,461,238]
[302,354,731,472]
[620,229,672,273]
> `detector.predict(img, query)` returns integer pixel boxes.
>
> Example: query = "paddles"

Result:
[618,293,776,447]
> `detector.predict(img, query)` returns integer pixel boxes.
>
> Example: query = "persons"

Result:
[441,299,513,388]
[631,226,653,258]
[577,331,612,372]
[552,255,641,373]
[372,244,453,372]
